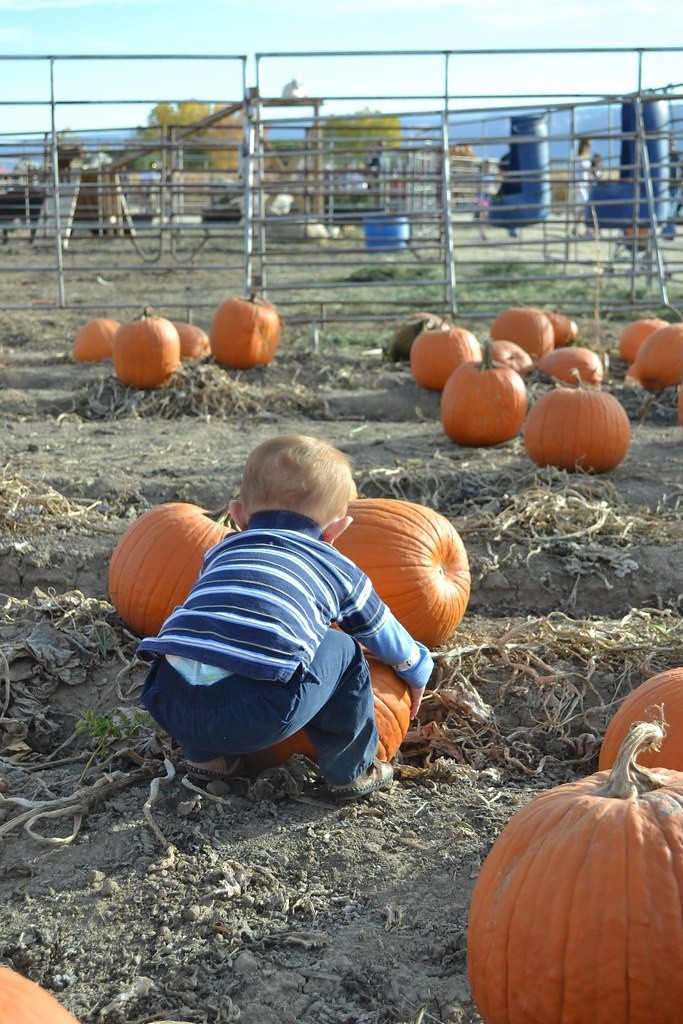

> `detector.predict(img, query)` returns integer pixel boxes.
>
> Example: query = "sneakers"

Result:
[184,754,247,780]
[327,759,395,799]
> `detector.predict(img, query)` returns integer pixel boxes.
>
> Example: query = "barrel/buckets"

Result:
[363,216,412,250]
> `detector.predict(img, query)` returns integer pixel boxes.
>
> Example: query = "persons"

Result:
[365,141,386,189]
[135,435,434,799]
[500,154,518,236]
[572,139,603,239]
[475,160,493,240]
[661,139,683,240]
[141,162,171,216]
[340,161,367,189]
